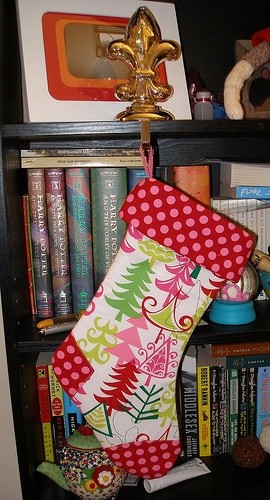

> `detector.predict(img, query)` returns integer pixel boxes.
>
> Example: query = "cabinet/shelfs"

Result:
[0,118,270,500]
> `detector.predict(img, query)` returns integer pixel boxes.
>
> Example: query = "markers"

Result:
[38,321,79,336]
[36,309,86,328]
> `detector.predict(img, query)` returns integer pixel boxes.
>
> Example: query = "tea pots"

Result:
[37,424,128,500]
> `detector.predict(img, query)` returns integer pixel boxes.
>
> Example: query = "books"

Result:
[175,342,270,465]
[18,351,83,478]
[5,138,270,332]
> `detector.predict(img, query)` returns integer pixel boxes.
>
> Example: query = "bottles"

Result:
[194,92,213,120]
